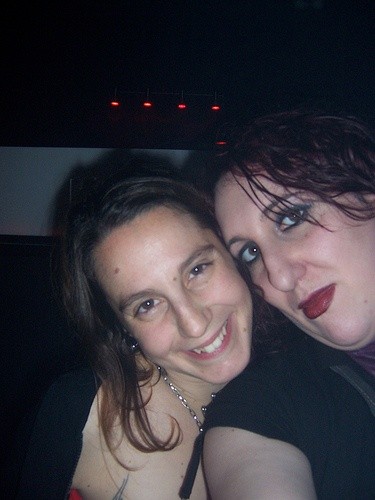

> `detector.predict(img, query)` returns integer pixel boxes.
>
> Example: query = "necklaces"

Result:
[155,363,218,433]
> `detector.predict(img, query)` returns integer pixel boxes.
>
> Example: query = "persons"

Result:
[13,155,300,500]
[199,94,375,500]
